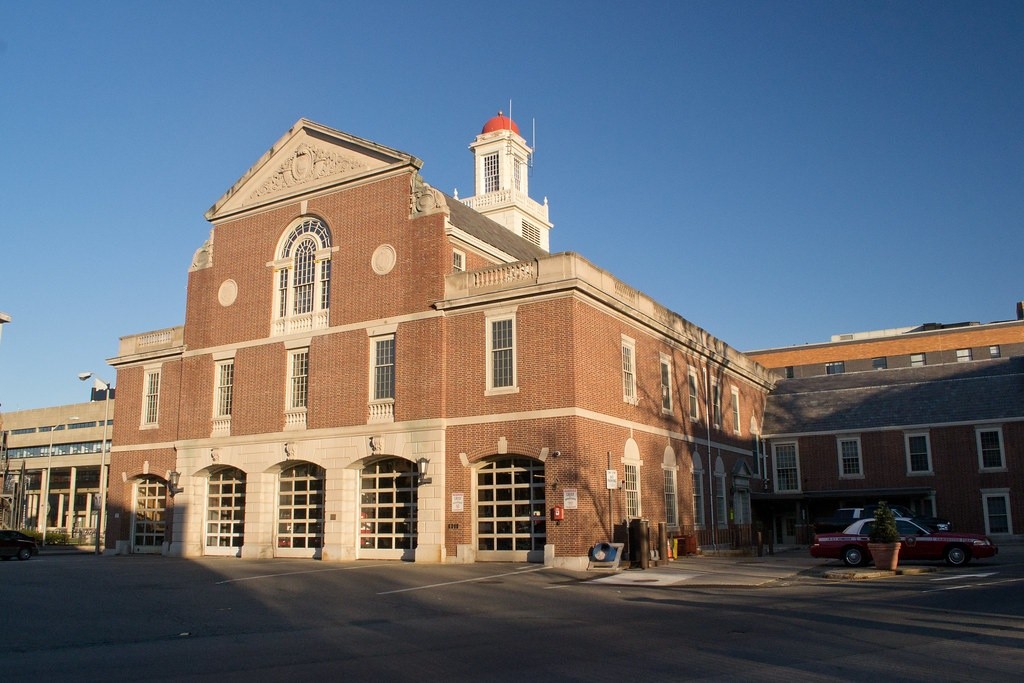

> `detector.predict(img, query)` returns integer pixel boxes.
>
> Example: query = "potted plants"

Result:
[867,500,901,571]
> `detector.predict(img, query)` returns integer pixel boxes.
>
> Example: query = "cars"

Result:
[813,506,952,534]
[0,530,36,561]
[809,517,999,567]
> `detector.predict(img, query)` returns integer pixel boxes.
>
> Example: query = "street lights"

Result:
[42,417,79,547]
[79,372,110,556]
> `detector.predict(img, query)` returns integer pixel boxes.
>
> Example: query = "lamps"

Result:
[416,457,432,486]
[170,472,183,494]
